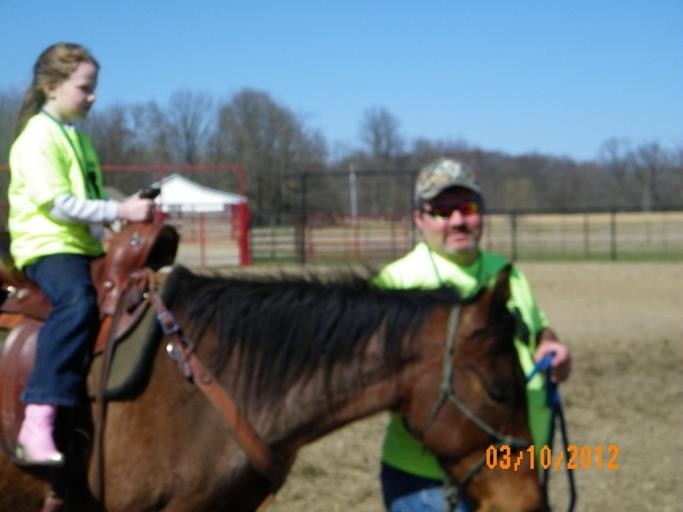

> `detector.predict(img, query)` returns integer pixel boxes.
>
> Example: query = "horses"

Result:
[0,261,548,511]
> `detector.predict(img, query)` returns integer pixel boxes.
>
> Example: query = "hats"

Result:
[414,156,482,210]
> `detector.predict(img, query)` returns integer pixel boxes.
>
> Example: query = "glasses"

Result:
[420,198,481,218]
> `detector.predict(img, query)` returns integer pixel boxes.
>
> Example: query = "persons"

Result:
[7,42,154,468]
[367,158,572,511]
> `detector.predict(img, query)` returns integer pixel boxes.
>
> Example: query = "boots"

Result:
[15,404,65,464]
